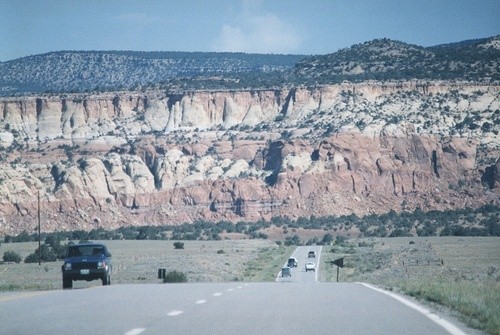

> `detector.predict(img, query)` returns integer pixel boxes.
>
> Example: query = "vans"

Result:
[281,267,292,277]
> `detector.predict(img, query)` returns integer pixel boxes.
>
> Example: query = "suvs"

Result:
[61,242,112,290]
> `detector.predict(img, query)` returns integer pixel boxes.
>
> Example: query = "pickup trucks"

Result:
[305,262,316,271]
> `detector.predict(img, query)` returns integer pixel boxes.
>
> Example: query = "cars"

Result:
[287,258,298,267]
[308,251,316,258]
